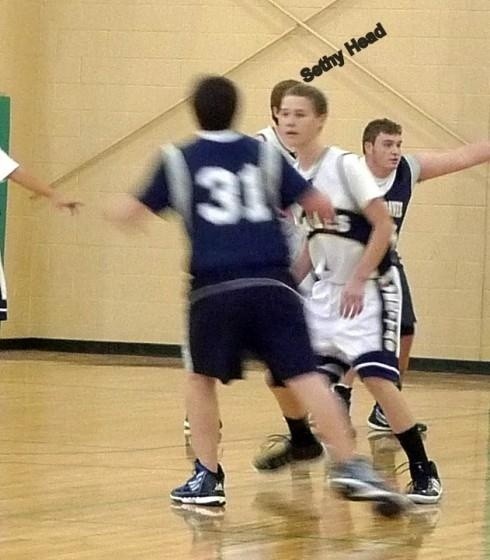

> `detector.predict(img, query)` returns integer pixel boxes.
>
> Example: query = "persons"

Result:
[169,430,439,558]
[249,83,445,510]
[101,75,419,522]
[0,148,86,322]
[183,76,308,435]
[333,118,489,429]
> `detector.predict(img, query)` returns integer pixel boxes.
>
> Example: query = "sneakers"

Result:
[183,417,222,437]
[253,433,327,474]
[169,458,226,506]
[325,403,443,511]
